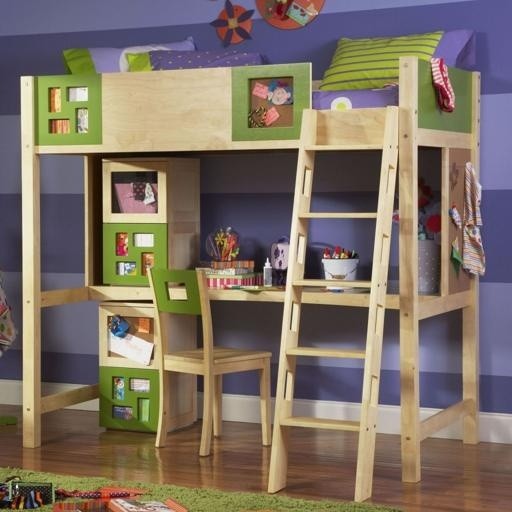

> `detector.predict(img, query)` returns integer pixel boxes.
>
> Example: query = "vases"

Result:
[417,241,441,295]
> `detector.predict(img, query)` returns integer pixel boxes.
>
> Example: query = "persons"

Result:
[117,234,127,256]
[116,378,124,398]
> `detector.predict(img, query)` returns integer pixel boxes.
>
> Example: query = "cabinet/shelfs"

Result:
[102,157,201,286]
[99,301,198,433]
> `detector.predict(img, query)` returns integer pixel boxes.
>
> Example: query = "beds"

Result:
[21,56,481,503]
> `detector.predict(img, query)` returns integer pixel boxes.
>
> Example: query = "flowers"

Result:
[393,176,441,239]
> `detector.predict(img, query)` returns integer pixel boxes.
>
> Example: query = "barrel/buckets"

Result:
[320,258,359,289]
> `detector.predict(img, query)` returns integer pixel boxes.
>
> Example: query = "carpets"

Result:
[0,467,405,512]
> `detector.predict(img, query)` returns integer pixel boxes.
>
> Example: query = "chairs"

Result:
[146,267,273,458]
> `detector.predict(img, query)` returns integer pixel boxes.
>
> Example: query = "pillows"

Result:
[126,49,263,71]
[318,29,472,93]
[62,35,196,75]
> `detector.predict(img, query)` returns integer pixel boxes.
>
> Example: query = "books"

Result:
[193,269,253,275]
[197,260,255,269]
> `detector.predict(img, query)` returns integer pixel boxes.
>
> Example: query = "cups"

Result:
[318,257,361,293]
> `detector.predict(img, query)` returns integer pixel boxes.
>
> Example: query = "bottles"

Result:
[263,257,272,288]
